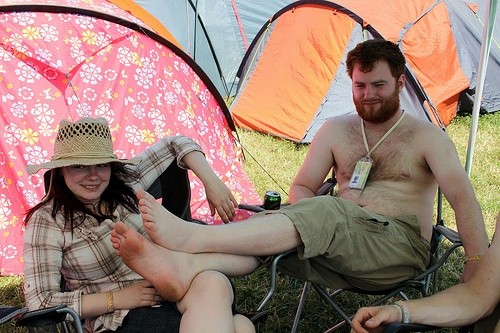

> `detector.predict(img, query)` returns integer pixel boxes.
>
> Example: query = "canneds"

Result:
[263,191,281,210]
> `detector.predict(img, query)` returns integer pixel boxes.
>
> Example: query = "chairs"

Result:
[0,169,500,333]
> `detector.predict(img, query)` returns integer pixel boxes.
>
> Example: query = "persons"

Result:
[110,38,489,302]
[22,116,256,333]
[349,210,500,333]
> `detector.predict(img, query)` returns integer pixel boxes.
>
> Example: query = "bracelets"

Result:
[395,300,410,324]
[106,290,114,312]
[464,256,486,264]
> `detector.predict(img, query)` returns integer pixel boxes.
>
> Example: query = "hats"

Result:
[24,116,144,175]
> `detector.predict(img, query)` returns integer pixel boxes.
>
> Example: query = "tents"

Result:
[0,0,500,276]
